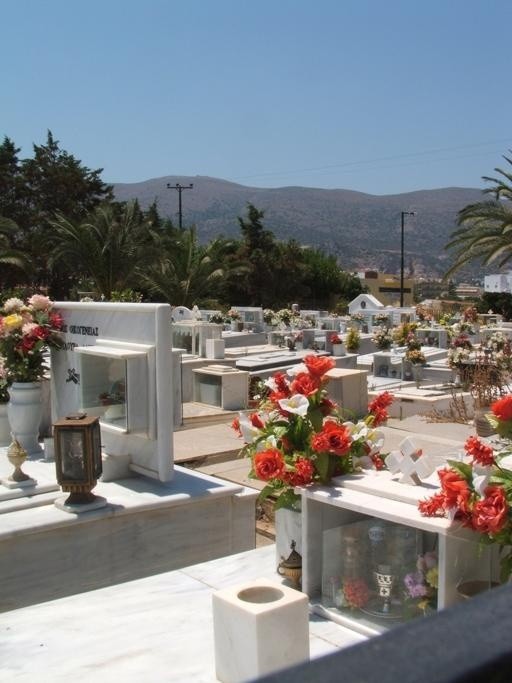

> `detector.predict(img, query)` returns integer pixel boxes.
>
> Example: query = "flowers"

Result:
[208,301,512,622]
[98,392,125,405]
[0,294,65,406]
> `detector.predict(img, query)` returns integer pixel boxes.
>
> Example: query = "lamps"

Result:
[52,413,103,505]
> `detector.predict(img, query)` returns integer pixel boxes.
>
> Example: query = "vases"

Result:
[6,381,45,455]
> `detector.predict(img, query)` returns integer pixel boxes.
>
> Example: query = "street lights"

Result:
[400,211,418,306]
